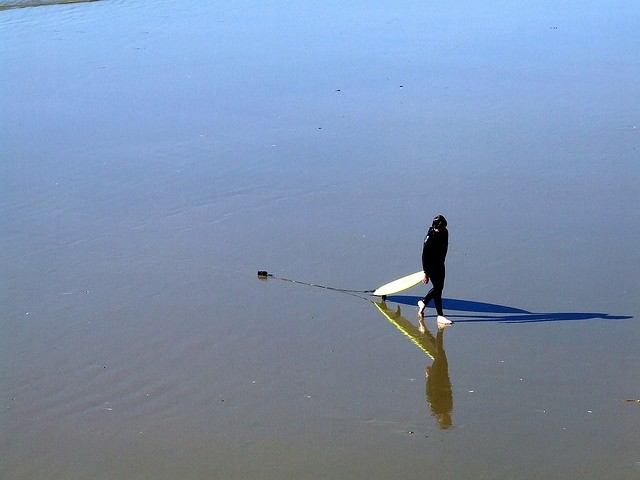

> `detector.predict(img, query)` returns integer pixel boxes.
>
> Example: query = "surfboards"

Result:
[373,271,426,301]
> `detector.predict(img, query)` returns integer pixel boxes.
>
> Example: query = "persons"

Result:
[418,319,453,429]
[418,215,453,324]
